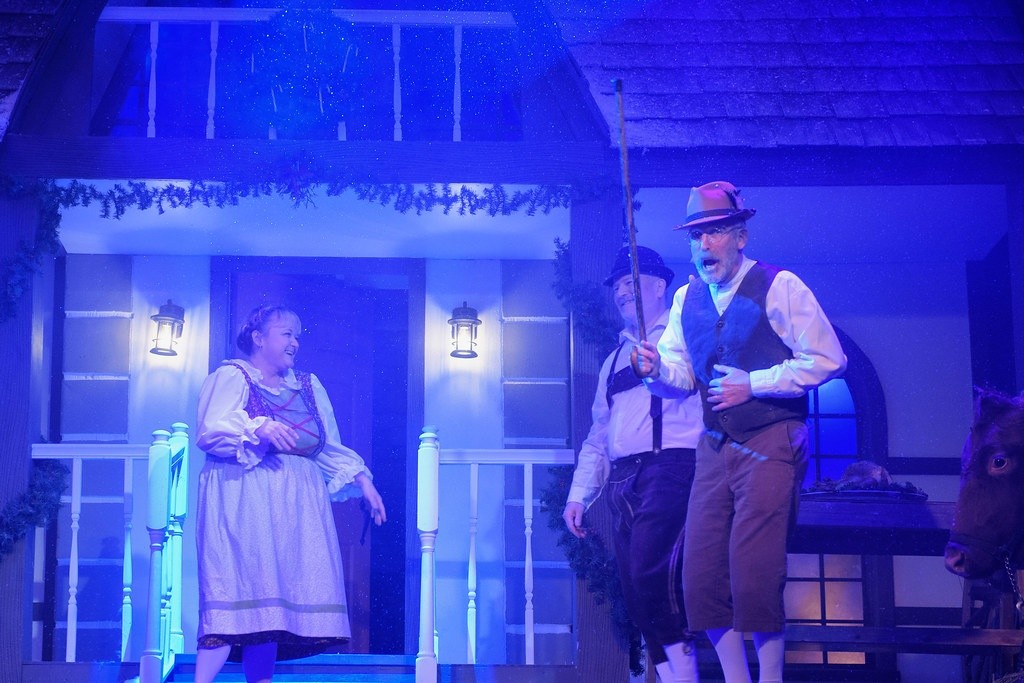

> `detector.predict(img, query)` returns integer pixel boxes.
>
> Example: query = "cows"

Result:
[944,386,1024,580]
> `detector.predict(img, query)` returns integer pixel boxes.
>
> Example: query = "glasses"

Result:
[684,225,744,246]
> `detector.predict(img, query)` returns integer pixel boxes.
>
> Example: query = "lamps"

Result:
[148,298,186,355]
[449,300,483,359]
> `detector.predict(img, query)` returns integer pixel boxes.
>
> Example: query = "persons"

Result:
[193,301,387,683]
[561,244,706,682]
[627,181,848,683]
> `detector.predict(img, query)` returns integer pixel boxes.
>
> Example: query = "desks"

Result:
[670,497,1024,683]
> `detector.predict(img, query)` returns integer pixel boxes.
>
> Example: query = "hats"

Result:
[673,180,757,231]
[601,246,674,289]
